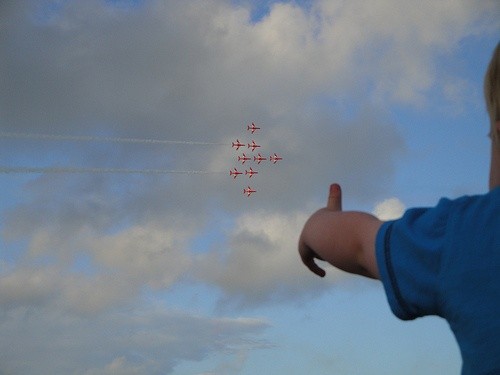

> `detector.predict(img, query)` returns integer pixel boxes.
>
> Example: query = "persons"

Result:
[298,43,500,375]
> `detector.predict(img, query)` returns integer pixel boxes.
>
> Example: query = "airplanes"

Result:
[229,120,286,197]
